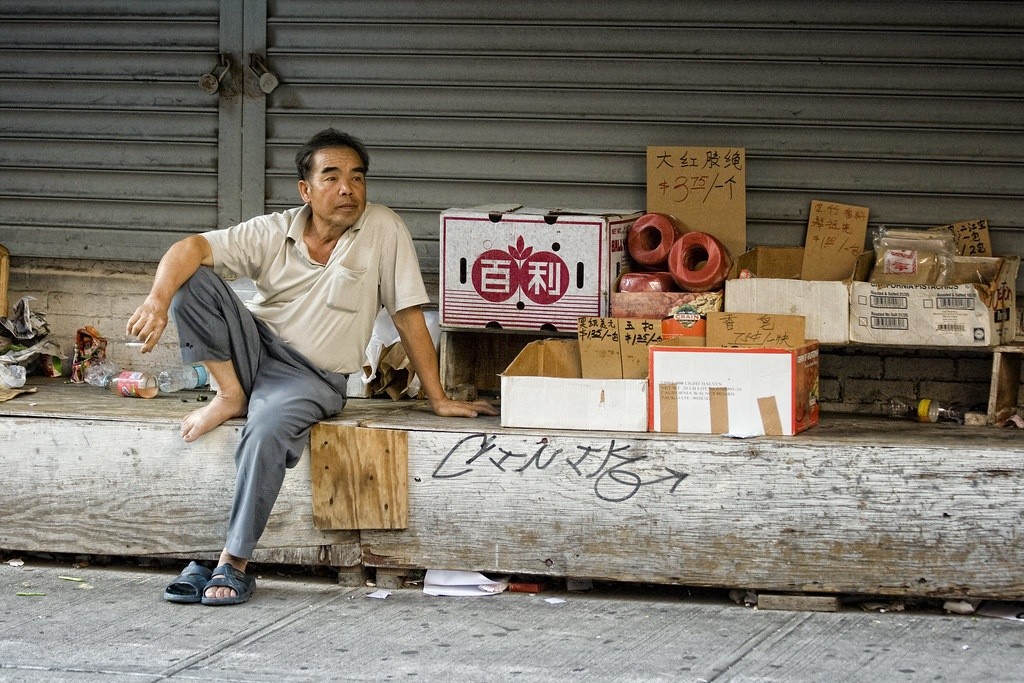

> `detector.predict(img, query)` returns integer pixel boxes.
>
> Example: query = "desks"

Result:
[440,329,1024,421]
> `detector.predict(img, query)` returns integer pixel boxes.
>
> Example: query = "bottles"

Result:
[98,370,158,399]
[158,364,210,392]
[885,395,960,424]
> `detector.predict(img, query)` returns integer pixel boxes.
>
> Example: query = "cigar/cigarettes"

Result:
[126,343,157,348]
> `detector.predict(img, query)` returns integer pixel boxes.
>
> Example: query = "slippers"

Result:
[202,563,257,605]
[163,561,213,602]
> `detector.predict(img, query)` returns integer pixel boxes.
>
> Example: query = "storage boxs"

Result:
[851,252,1020,346]
[726,248,852,346]
[610,272,725,318]
[501,340,649,432]
[438,203,644,330]
[649,336,821,437]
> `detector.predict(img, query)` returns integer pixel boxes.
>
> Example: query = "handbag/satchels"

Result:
[361,306,444,402]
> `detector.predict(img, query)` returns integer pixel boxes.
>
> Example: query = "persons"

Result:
[126,128,500,606]
